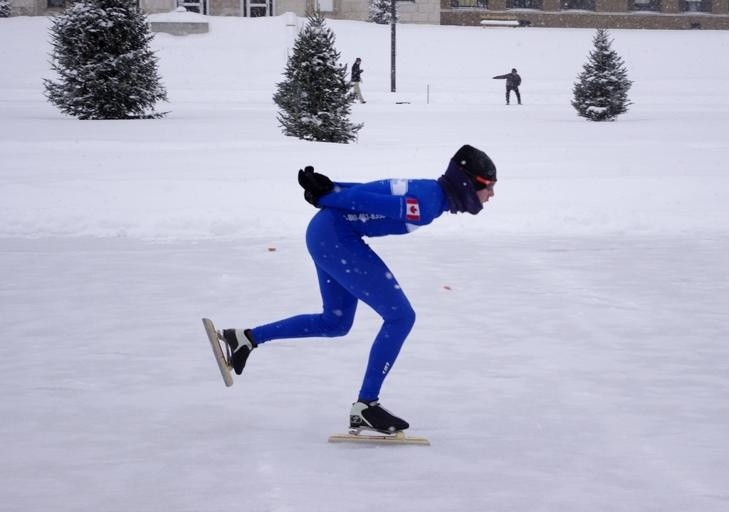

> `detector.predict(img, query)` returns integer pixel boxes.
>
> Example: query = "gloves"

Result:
[295,162,335,209]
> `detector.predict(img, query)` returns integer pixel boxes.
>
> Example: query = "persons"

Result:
[491,68,522,105]
[222,141,499,434]
[348,58,368,104]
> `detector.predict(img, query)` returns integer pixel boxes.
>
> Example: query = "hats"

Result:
[450,145,497,192]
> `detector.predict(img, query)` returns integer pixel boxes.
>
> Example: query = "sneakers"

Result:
[222,327,255,375]
[349,399,410,434]
